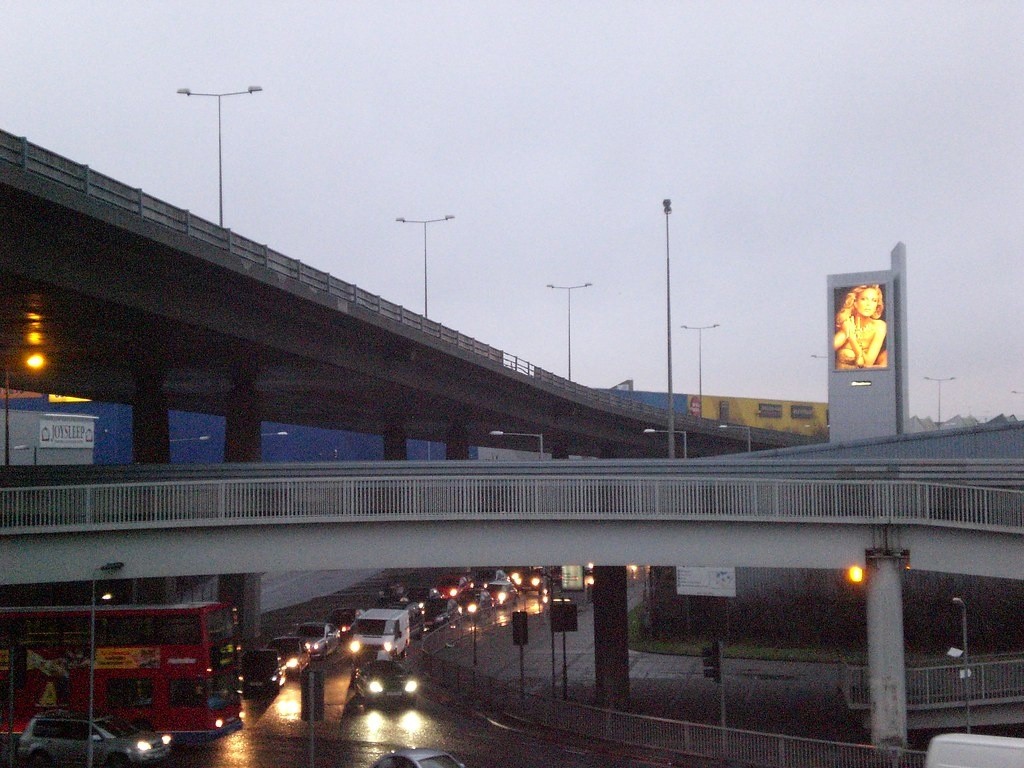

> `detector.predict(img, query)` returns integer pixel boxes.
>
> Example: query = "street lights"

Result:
[952,598,970,735]
[547,283,593,380]
[176,85,262,226]
[396,215,454,319]
[489,431,543,461]
[87,563,125,768]
[644,429,687,458]
[720,424,750,453]
[681,325,720,418]
[925,377,957,429]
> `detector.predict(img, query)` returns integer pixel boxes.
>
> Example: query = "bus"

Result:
[0,602,244,743]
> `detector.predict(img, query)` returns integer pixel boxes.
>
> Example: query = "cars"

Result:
[244,650,286,691]
[353,660,417,702]
[290,623,340,660]
[326,609,360,634]
[349,569,546,658]
[263,637,310,675]
[368,749,465,768]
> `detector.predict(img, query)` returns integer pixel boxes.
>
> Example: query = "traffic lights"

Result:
[703,639,721,683]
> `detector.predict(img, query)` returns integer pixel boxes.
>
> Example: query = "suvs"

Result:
[18,711,171,768]
